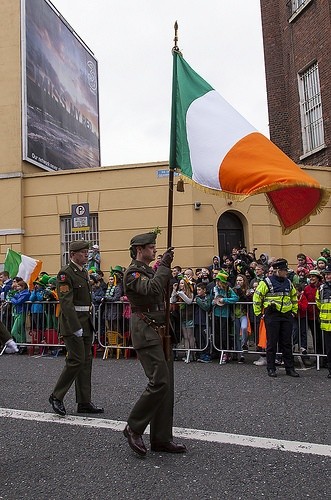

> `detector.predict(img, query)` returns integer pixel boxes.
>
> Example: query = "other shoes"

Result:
[222,353,232,363]
[242,346,249,352]
[238,358,246,364]
[253,357,267,366]
[197,354,212,363]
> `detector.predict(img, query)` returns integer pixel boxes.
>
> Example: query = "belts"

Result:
[74,306,91,312]
[131,303,165,312]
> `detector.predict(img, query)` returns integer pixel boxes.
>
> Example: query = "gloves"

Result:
[74,328,83,337]
[160,246,174,266]
[5,340,19,353]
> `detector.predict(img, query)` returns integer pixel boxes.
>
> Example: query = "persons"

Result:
[47,240,104,416]
[169,246,331,380]
[153,255,163,272]
[123,233,187,456]
[88,244,131,360]
[0,271,64,356]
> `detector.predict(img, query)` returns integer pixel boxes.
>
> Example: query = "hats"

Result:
[213,270,229,283]
[324,264,331,272]
[69,240,89,251]
[129,232,157,246]
[90,272,99,282]
[32,274,57,288]
[309,270,321,276]
[271,260,289,270]
[110,265,124,276]
[317,256,328,264]
[321,247,330,256]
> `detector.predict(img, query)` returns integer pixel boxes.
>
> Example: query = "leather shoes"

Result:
[268,368,277,377]
[286,369,299,377]
[77,402,104,413]
[151,441,188,453]
[123,424,147,456]
[49,393,66,415]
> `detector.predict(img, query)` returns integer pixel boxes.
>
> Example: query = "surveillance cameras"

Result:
[195,201,201,210]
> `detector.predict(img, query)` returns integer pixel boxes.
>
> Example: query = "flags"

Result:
[5,249,42,290]
[174,51,331,235]
